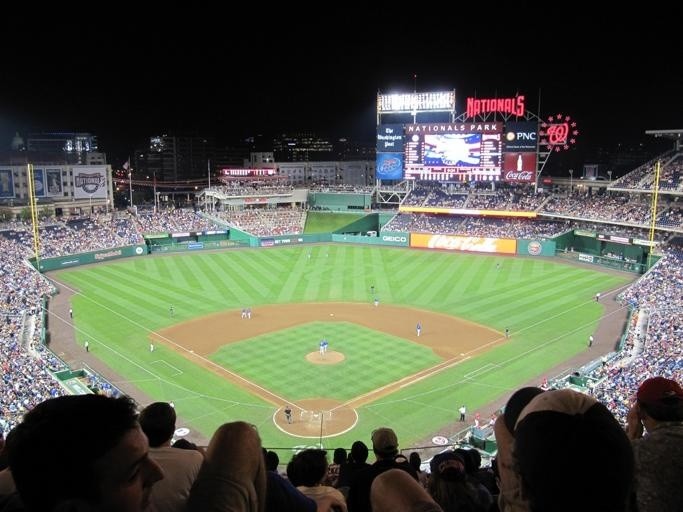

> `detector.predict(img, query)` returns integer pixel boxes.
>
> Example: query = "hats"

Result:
[636,376,683,408]
[504,386,635,500]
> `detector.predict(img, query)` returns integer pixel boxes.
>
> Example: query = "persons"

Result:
[0,257,95,372]
[1,173,574,257]
[415,320,421,337]
[307,251,310,260]
[323,336,327,352]
[168,305,174,320]
[496,260,501,272]
[319,339,323,356]
[374,297,378,307]
[247,305,252,321]
[576,153,682,373]
[241,306,246,320]
[1,373,683,511]
[505,326,510,341]
[150,338,154,352]
[370,284,374,296]
